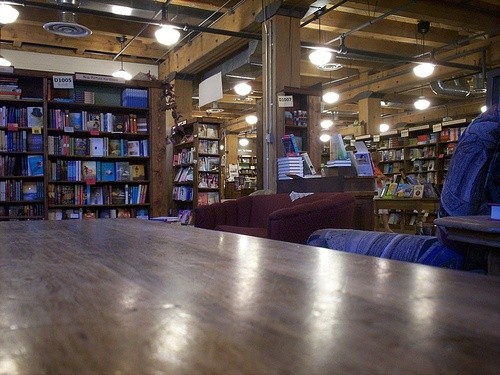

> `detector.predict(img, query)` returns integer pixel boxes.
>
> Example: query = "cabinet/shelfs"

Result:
[236,155,259,190]
[167,116,223,224]
[353,117,476,236]
[278,87,314,176]
[0,67,150,222]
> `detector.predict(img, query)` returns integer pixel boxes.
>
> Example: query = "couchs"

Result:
[193,191,363,244]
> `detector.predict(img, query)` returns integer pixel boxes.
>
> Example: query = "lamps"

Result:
[320,107,333,129]
[43,11,93,38]
[379,117,389,133]
[308,16,333,68]
[319,130,331,143]
[322,71,340,104]
[412,20,435,78]
[0,0,20,25]
[154,3,181,46]
[245,115,258,125]
[110,37,133,81]
[414,80,431,111]
[234,83,252,96]
[239,138,249,147]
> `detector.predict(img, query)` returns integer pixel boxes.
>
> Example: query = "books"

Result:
[0,154,145,180]
[0,76,148,110]
[0,129,149,157]
[150,119,221,221]
[0,180,149,220]
[347,123,468,237]
[0,104,148,134]
[489,203,500,221]
[276,111,316,180]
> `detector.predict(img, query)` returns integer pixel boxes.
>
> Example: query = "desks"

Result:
[0,217,499,375]
[372,196,443,236]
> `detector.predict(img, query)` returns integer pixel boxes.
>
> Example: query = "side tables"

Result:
[432,214,500,278]
